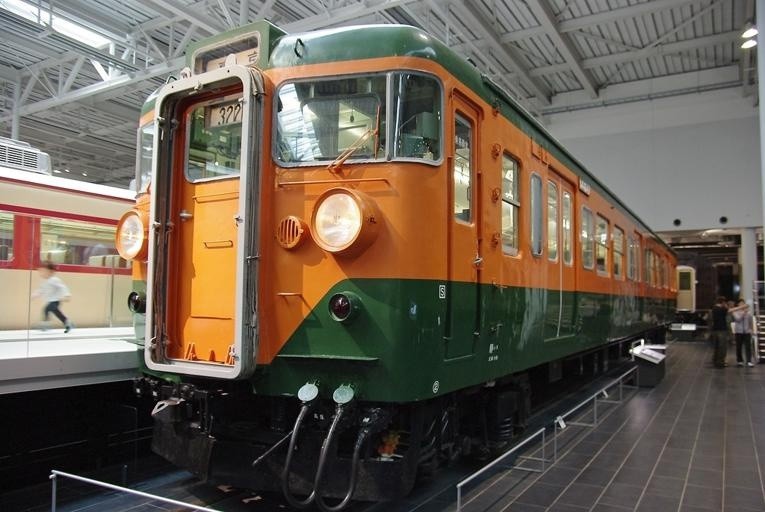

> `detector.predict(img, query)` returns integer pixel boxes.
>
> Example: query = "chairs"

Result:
[38,249,127,269]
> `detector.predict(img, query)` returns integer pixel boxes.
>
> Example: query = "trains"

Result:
[0,132,137,333]
[113,18,680,510]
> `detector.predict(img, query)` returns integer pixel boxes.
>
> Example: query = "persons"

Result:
[31,265,73,333]
[733,300,755,367]
[712,296,749,368]
[726,301,736,344]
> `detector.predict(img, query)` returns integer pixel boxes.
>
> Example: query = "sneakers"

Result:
[737,362,755,367]
